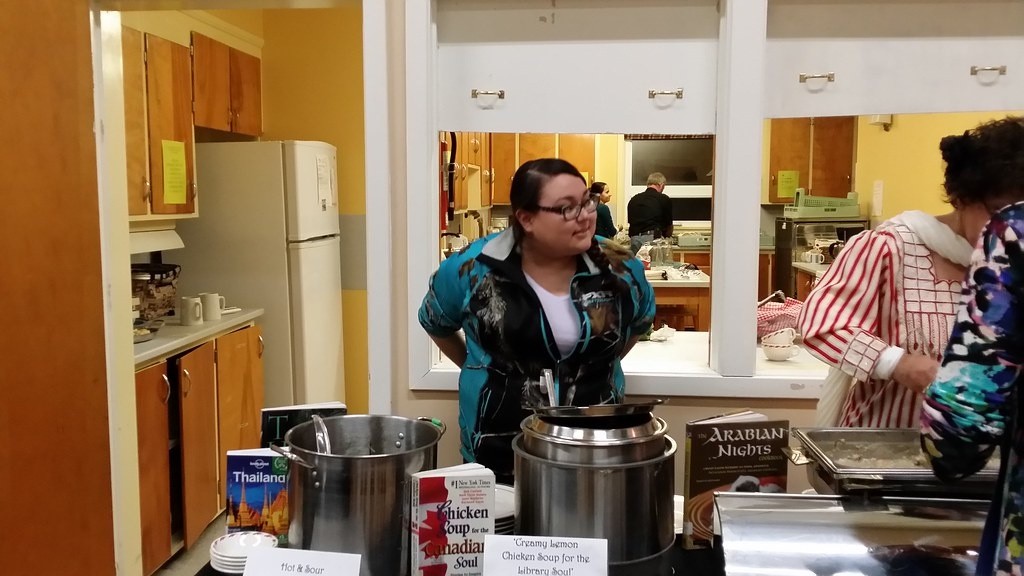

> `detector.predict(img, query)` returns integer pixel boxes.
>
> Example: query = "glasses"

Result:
[535,192,600,221]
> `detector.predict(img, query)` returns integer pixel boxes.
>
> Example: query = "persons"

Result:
[418,159,655,484]
[628,172,673,256]
[921,200,1024,575]
[798,116,1024,428]
[591,182,623,239]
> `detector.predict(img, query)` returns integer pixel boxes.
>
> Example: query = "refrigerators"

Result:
[161,140,345,405]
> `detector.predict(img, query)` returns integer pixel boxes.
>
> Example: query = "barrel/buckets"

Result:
[273,415,446,576]
[511,415,677,576]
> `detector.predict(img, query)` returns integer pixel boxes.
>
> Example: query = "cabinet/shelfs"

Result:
[135,341,222,575]
[481,133,490,206]
[448,132,469,212]
[762,116,858,207]
[216,323,268,513]
[123,24,197,218]
[557,132,594,186]
[188,32,264,139]
[492,133,558,204]
[467,133,480,166]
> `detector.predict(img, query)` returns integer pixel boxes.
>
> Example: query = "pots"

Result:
[133,318,165,341]
[521,395,663,423]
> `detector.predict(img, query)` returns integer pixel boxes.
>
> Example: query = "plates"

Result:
[209,531,279,574]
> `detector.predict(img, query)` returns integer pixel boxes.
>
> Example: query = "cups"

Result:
[761,327,796,344]
[180,295,203,325]
[811,253,825,264]
[198,292,225,320]
[762,343,800,362]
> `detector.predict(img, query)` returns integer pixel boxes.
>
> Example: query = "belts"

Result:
[633,231,654,235]
